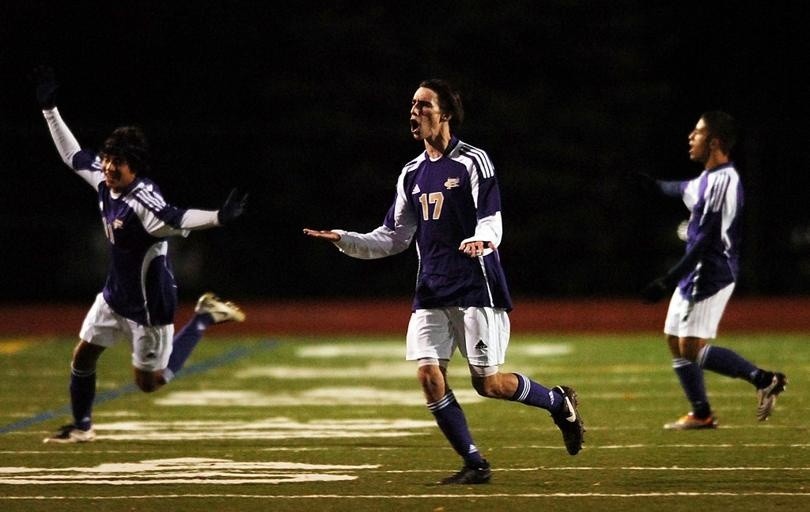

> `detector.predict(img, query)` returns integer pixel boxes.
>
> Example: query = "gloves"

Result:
[23,62,61,111]
[638,265,680,305]
[218,187,250,227]
[636,171,662,198]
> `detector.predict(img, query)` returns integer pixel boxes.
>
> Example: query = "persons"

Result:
[302,78,586,487]
[641,110,789,434]
[26,64,252,448]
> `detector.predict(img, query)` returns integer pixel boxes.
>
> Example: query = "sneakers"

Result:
[193,291,247,326]
[663,411,720,430]
[550,383,587,456]
[41,419,97,445]
[755,371,789,420]
[432,459,496,485]
